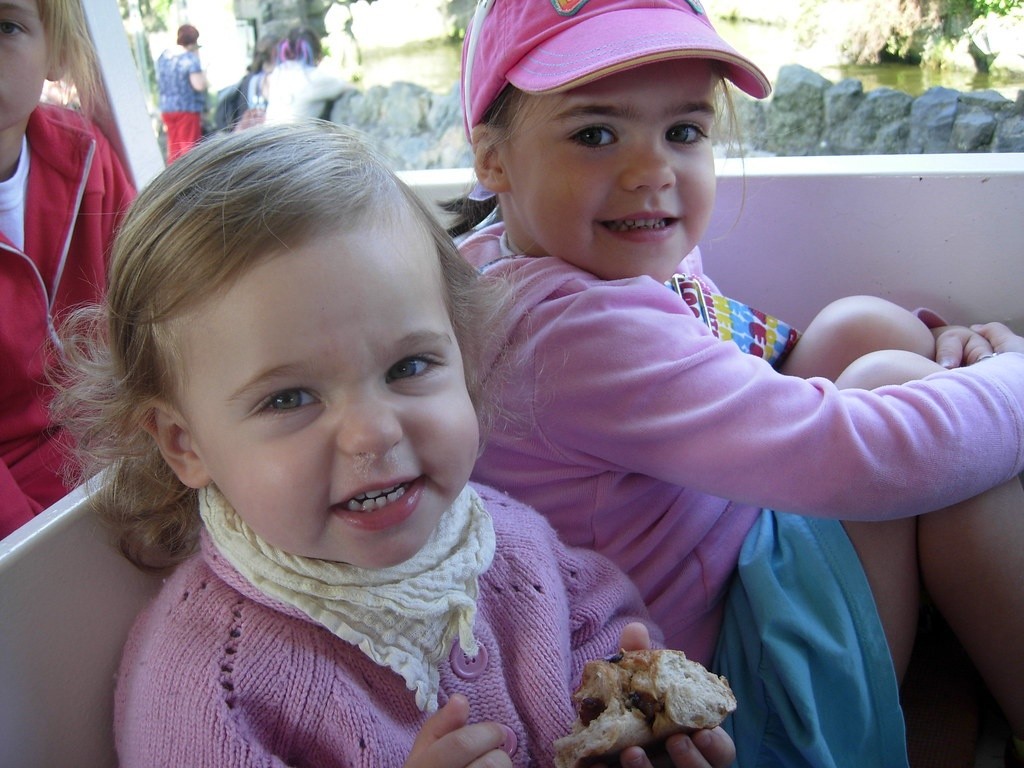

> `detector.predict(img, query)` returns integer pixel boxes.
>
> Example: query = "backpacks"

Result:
[214,87,240,133]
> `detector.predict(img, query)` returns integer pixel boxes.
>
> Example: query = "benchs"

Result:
[0,221,523,768]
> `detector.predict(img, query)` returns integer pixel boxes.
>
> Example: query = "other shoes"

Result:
[1004,734,1024,768]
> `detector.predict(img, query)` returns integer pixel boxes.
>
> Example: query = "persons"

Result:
[51,121,736,768]
[234,21,315,132]
[154,22,207,164]
[445,0,1024,768]
[0,0,136,541]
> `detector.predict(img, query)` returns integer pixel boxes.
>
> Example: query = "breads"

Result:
[552,648,738,768]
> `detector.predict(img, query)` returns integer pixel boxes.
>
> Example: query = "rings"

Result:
[978,352,997,361]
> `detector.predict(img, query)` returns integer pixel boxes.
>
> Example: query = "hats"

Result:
[460,0,772,145]
